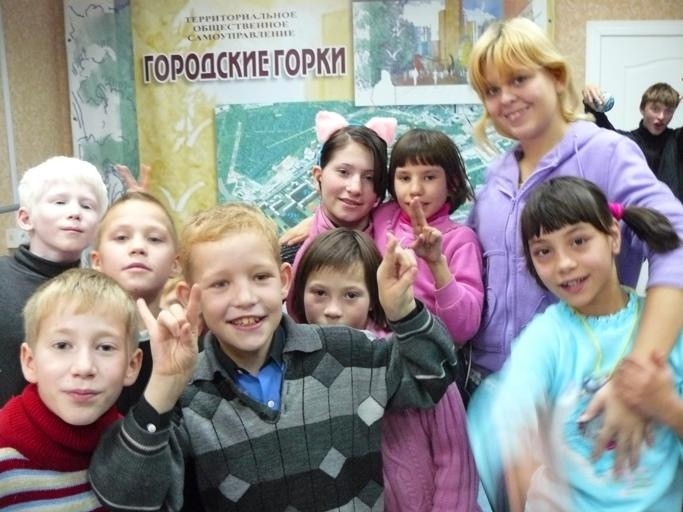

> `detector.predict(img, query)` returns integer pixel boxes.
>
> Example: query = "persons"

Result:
[466,176,683,512]
[582,82,683,206]
[463,21,683,476]
[91,193,179,415]
[291,228,479,511]
[280,124,484,341]
[91,204,458,512]
[1,269,144,512]
[0,156,150,407]
